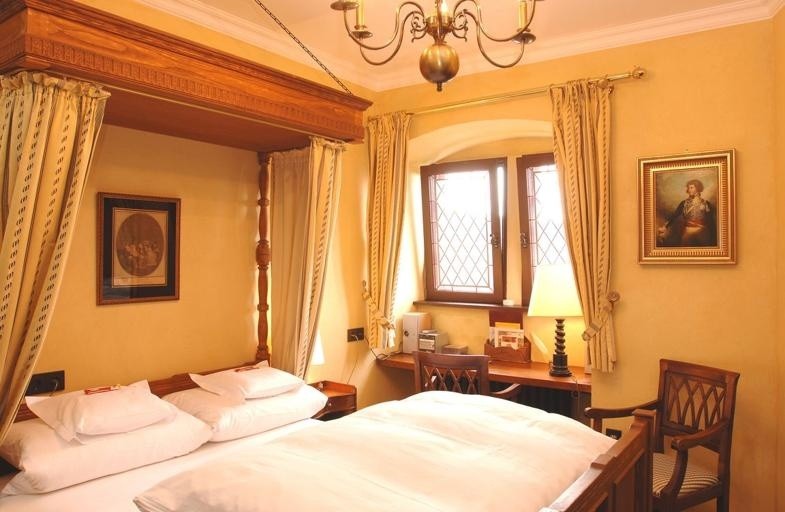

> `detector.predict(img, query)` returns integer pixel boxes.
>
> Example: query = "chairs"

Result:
[412,350,521,402]
[582,356,740,511]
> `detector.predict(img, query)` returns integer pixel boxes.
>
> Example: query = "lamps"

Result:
[331,0,539,93]
[309,328,325,368]
[526,261,585,378]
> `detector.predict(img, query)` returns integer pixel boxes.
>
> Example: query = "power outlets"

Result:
[346,328,366,342]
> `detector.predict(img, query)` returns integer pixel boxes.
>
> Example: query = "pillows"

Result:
[160,382,328,446]
[25,377,177,445]
[0,398,216,495]
[191,358,306,404]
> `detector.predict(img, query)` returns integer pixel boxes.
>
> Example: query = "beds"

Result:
[0,0,658,510]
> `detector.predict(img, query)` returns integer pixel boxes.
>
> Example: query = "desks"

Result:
[377,350,592,422]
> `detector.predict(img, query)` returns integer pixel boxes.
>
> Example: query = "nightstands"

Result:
[306,378,359,422]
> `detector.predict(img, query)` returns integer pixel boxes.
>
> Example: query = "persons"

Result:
[657,179,718,246]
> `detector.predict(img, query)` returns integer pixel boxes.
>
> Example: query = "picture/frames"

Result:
[93,190,183,309]
[634,147,740,271]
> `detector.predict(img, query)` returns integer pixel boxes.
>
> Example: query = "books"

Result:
[488,322,524,349]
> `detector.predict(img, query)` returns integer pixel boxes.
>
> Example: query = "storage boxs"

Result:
[482,336,531,364]
